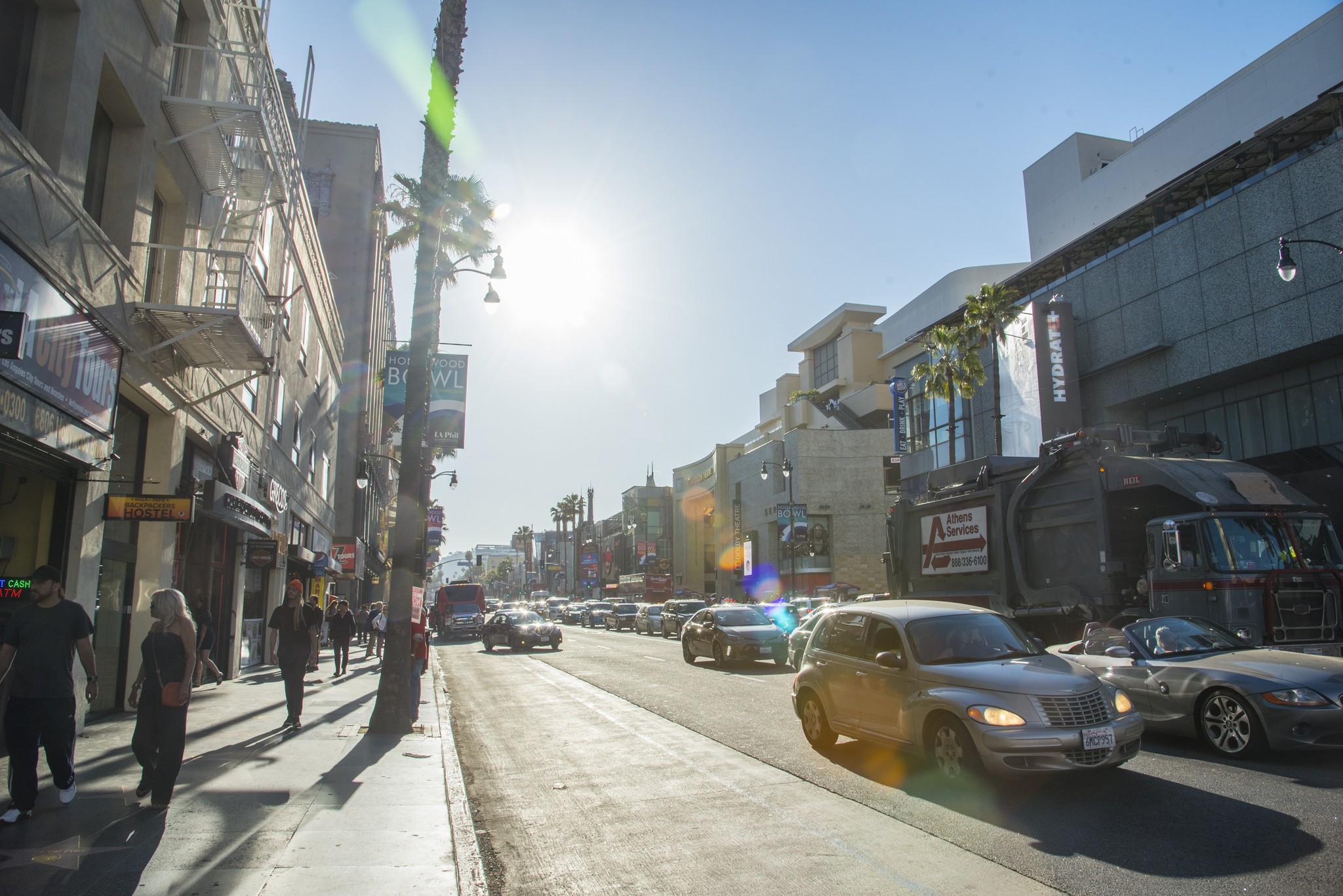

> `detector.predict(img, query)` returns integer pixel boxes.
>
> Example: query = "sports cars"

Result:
[1044,613,1343,760]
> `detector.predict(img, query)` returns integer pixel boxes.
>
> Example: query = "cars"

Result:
[481,589,892,668]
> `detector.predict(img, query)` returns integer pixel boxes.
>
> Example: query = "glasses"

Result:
[200,595,204,600]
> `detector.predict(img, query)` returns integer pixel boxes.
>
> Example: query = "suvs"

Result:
[792,597,1145,794]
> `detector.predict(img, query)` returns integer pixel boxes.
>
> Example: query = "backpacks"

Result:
[365,617,376,632]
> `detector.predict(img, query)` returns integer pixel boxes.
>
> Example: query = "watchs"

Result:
[87,675,98,682]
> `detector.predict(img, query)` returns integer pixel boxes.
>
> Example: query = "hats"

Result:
[26,565,63,584]
[287,579,303,592]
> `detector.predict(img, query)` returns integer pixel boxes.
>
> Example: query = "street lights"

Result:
[506,566,515,583]
[586,532,603,601]
[761,457,814,600]
[523,560,538,594]
[627,515,648,574]
[548,549,566,597]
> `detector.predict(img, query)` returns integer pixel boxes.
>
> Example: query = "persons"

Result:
[128,587,197,810]
[1154,626,1192,655]
[365,601,383,657]
[706,593,856,605]
[411,612,427,723]
[357,605,369,645]
[325,599,339,648]
[371,604,389,664]
[308,594,324,664]
[807,523,829,555]
[0,565,99,824]
[192,596,224,688]
[329,600,357,676]
[1261,523,1311,569]
[887,632,920,659]
[336,600,389,621]
[421,603,437,632]
[370,603,377,656]
[933,629,972,660]
[304,602,319,672]
[266,579,318,727]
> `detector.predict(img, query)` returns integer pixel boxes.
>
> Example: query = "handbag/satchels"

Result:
[159,681,189,708]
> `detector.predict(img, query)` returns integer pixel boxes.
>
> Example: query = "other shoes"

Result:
[358,641,361,645]
[379,660,384,664]
[377,655,383,658]
[364,640,368,644]
[366,652,376,656]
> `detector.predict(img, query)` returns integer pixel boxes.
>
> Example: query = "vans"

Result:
[434,579,486,642]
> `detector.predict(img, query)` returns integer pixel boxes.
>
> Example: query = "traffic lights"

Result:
[469,577,472,583]
[540,560,545,569]
[490,578,494,587]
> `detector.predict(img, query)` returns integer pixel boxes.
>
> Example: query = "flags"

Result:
[426,353,468,449]
[379,350,410,445]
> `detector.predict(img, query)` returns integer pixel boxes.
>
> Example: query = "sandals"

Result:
[191,681,201,687]
[216,672,224,686]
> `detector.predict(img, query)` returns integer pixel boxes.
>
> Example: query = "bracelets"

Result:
[310,657,318,659]
[131,681,141,691]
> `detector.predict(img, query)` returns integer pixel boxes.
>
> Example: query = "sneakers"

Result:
[0,803,32,823]
[151,802,168,810]
[341,670,346,674]
[292,715,302,727]
[283,715,293,725]
[59,782,77,804]
[135,768,160,797]
[333,672,339,676]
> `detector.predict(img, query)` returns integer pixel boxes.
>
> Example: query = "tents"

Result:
[820,581,861,595]
[672,588,702,600]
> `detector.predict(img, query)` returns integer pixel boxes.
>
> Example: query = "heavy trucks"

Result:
[881,419,1342,654]
